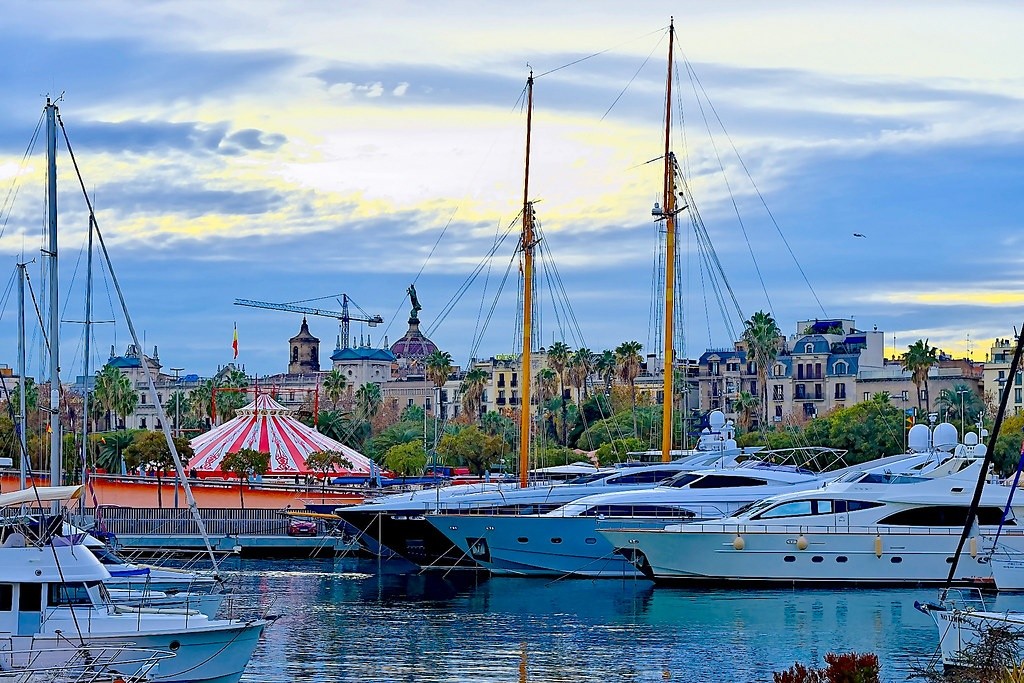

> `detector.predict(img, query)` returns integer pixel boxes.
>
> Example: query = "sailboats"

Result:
[0,14,1024,682]
